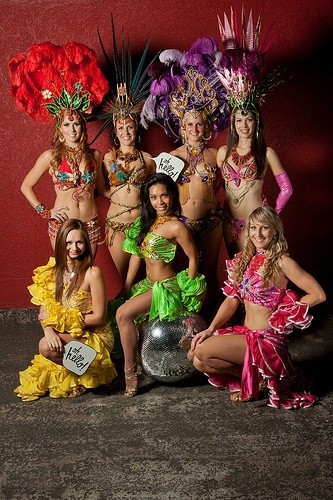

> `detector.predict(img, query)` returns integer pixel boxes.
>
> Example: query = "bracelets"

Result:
[34,204,51,218]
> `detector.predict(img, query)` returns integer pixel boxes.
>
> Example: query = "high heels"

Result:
[230,377,268,403]
[124,362,148,398]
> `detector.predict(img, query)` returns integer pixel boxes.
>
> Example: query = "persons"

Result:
[7,5,327,410]
[117,173,208,398]
[13,219,117,402]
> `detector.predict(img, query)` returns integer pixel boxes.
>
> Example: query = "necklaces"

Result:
[61,258,80,287]
[230,143,253,167]
[116,149,139,168]
[62,143,85,176]
[245,245,267,280]
[147,212,174,234]
[183,142,205,172]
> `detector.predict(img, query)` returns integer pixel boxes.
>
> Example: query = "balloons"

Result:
[137,314,208,384]
